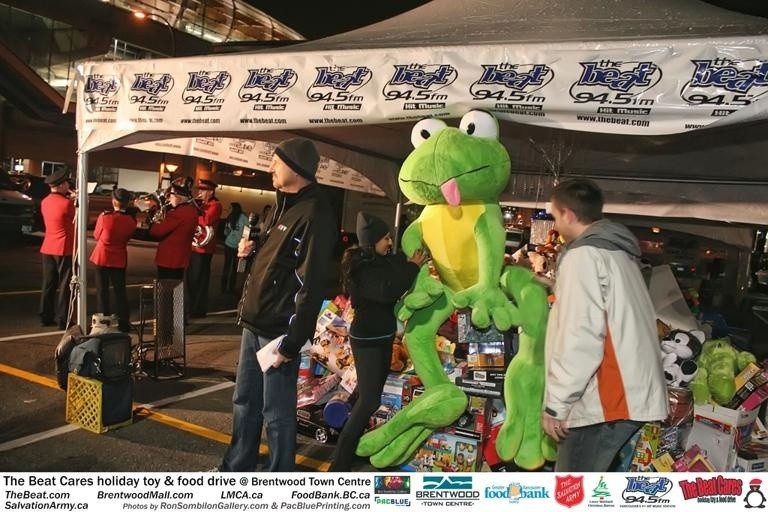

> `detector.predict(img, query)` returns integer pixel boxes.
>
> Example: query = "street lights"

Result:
[130,8,178,58]
[163,161,180,190]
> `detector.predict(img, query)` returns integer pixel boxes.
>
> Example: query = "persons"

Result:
[355,108,559,469]
[639,256,653,290]
[540,179,669,471]
[188,178,222,319]
[329,211,429,473]
[39,166,76,330]
[88,188,138,333]
[148,183,199,346]
[223,202,248,293]
[202,136,341,471]
[255,204,272,229]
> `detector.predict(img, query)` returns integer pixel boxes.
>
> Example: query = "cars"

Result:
[634,229,767,330]
[516,219,525,226]
[0,163,159,247]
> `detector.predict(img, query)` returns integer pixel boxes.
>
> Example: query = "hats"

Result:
[355,211,392,247]
[170,182,192,198]
[274,136,321,182]
[192,179,218,191]
[112,188,132,203]
[44,168,73,188]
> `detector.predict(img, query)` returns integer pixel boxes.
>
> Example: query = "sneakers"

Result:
[58,319,77,331]
[39,319,58,327]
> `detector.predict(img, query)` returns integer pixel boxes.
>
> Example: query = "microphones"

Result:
[237,211,260,272]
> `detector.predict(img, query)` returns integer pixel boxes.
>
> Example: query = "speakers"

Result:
[69,333,131,380]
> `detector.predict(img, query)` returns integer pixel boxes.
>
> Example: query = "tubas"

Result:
[165,176,214,247]
[135,188,171,227]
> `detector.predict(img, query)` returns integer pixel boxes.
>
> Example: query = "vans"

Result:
[503,226,525,255]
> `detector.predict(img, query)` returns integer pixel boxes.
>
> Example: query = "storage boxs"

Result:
[693,403,761,448]
